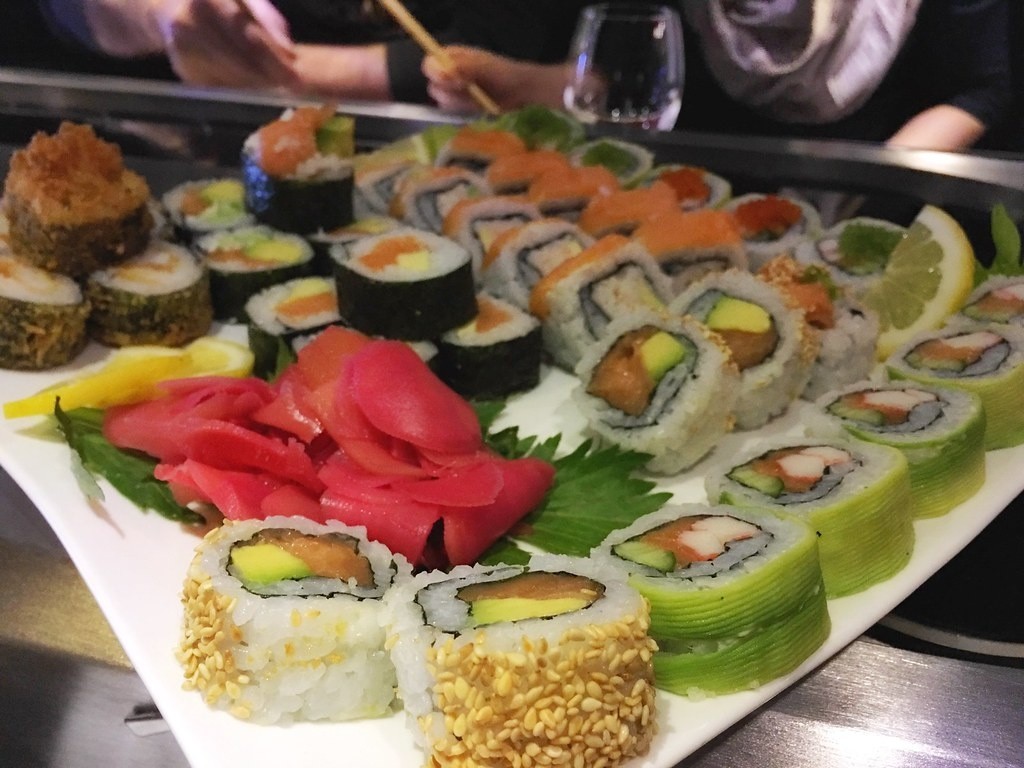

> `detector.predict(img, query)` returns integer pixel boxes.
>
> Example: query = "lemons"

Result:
[875,203,974,360]
[1,339,255,419]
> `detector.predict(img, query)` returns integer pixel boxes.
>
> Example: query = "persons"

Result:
[419,0,1024,157]
[25,1,585,113]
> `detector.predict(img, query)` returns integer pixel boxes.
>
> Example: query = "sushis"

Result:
[0,102,1024,768]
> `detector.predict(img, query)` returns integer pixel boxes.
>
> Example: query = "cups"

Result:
[564,6,686,148]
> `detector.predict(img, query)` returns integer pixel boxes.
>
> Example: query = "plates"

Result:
[0,321,1024,768]
